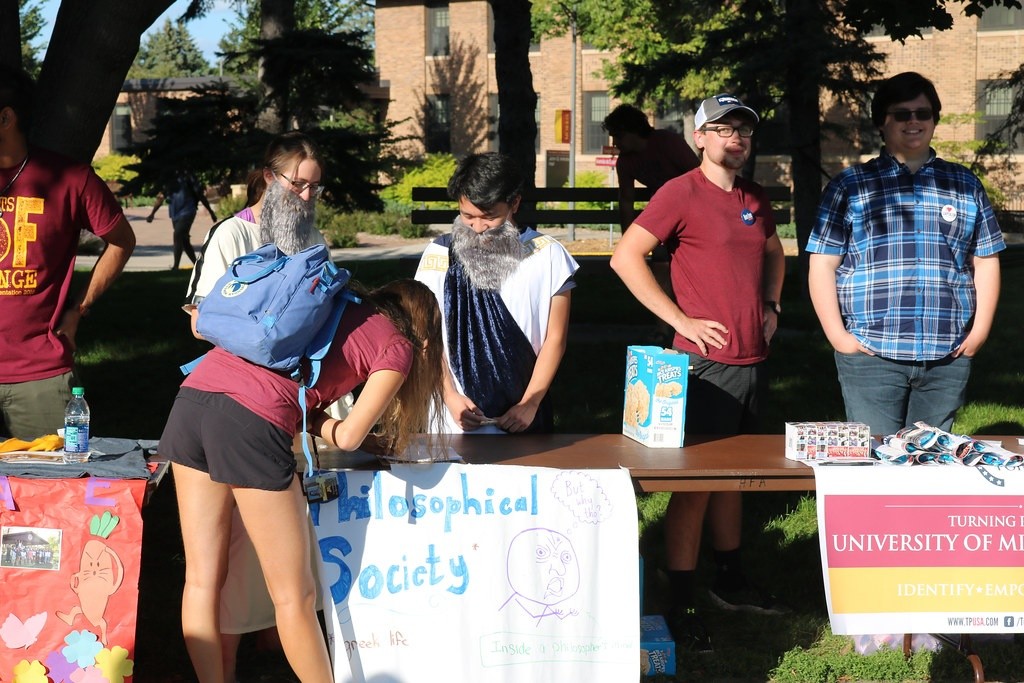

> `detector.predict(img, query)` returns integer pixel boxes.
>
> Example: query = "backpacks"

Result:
[196,244,394,390]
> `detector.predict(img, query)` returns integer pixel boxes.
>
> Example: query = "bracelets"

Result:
[75,303,90,316]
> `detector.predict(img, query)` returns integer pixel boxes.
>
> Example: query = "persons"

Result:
[182,131,323,683]
[413,151,580,434]
[806,71,1006,436]
[609,92,786,653]
[603,103,701,342]
[146,170,217,270]
[0,68,136,442]
[158,277,444,683]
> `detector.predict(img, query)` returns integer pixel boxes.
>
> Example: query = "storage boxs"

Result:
[622,345,690,449]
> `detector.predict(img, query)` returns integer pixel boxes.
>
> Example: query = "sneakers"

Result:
[664,608,714,654]
[708,579,794,616]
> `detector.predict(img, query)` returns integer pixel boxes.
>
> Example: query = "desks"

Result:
[291,434,1024,683]
[0,438,172,683]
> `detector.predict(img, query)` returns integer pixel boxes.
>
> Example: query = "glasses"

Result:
[884,110,934,121]
[700,126,755,138]
[265,165,325,196]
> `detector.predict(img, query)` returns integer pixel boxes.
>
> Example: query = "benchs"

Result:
[414,183,800,345]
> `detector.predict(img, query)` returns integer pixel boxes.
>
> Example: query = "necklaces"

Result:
[0,156,29,217]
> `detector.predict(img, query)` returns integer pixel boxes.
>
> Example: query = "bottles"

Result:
[62,387,88,464]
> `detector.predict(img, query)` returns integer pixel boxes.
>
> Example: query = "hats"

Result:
[694,93,759,131]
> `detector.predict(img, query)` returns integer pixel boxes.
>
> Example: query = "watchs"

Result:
[765,300,781,315]
